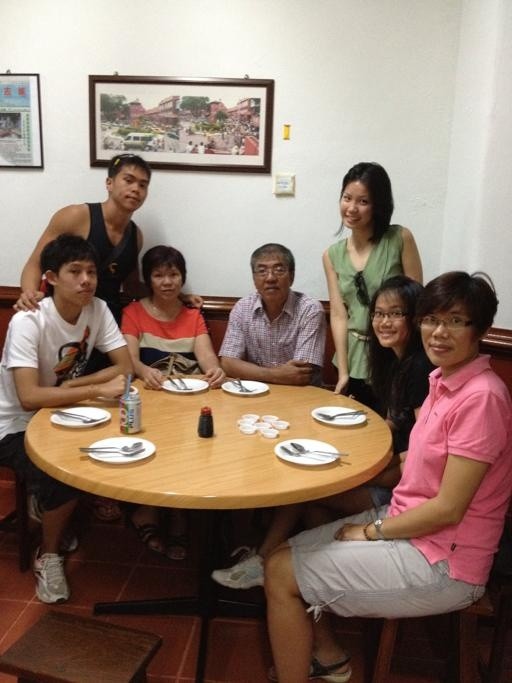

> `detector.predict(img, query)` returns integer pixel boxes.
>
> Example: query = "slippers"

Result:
[97,502,187,560]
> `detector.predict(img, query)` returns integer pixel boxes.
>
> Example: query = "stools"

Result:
[371,592,493,682]
[0,610,163,682]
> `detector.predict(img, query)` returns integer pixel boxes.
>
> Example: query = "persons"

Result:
[264,271,511,683]
[211,274,438,589]
[122,244,227,562]
[13,153,205,523]
[322,163,423,402]
[0,235,136,605]
[219,243,328,524]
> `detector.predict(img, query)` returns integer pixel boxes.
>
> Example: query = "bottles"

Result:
[197,405,213,438]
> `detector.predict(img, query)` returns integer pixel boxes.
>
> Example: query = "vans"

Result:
[123,132,153,149]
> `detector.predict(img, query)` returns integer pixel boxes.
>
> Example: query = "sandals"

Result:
[268,649,352,683]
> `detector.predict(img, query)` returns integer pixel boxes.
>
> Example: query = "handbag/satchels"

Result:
[151,352,202,376]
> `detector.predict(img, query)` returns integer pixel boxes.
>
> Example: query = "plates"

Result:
[50,405,111,428]
[220,379,269,396]
[310,406,368,426]
[273,438,338,466]
[162,377,209,393]
[88,436,156,464]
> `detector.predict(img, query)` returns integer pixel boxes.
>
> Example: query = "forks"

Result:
[281,446,328,461]
[51,410,107,423]
[88,448,146,457]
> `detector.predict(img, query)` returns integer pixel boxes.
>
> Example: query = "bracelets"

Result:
[363,520,374,540]
[89,384,94,401]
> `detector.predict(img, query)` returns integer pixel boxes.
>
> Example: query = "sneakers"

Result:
[212,547,265,590]
[29,494,78,604]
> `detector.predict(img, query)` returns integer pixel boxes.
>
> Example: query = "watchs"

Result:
[375,518,383,541]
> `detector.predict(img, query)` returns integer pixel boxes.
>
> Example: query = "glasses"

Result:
[419,315,472,329]
[354,272,369,305]
[371,311,407,320]
[252,266,290,276]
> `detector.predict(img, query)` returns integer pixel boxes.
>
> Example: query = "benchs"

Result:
[0,286,512,682]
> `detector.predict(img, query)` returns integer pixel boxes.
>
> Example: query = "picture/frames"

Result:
[89,75,275,173]
[0,74,44,168]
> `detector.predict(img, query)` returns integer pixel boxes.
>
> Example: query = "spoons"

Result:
[315,410,368,421]
[290,441,349,457]
[79,442,143,450]
